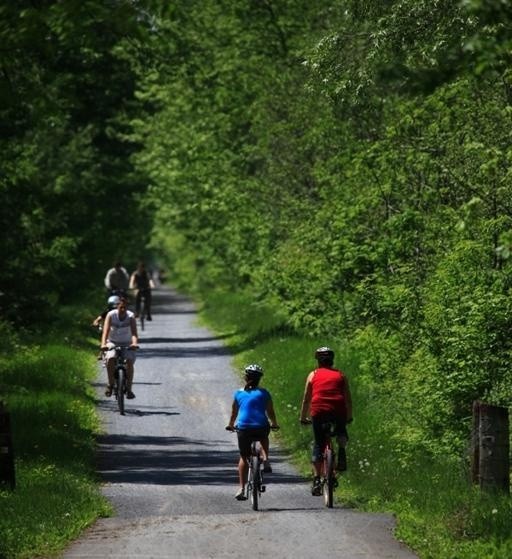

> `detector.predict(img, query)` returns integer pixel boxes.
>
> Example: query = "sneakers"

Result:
[263,460,272,473]
[312,477,321,496]
[235,490,245,500]
[338,447,346,471]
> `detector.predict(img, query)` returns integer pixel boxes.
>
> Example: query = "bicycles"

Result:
[101,346,139,415]
[107,287,128,301]
[225,425,280,511]
[129,286,157,331]
[300,417,354,509]
[94,323,110,369]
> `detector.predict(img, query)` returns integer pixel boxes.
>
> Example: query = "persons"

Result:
[101,297,138,399]
[93,295,121,365]
[229,364,278,498]
[129,263,153,322]
[299,346,354,497]
[104,261,129,294]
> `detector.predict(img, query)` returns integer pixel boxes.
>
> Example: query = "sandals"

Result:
[125,388,135,399]
[105,385,113,397]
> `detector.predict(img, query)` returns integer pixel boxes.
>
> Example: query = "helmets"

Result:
[315,347,334,359]
[107,295,121,308]
[244,364,263,375]
